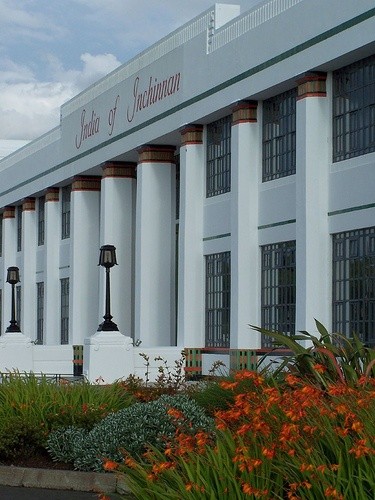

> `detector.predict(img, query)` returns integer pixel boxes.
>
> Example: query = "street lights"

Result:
[98,245,120,331]
[5,267,22,333]
